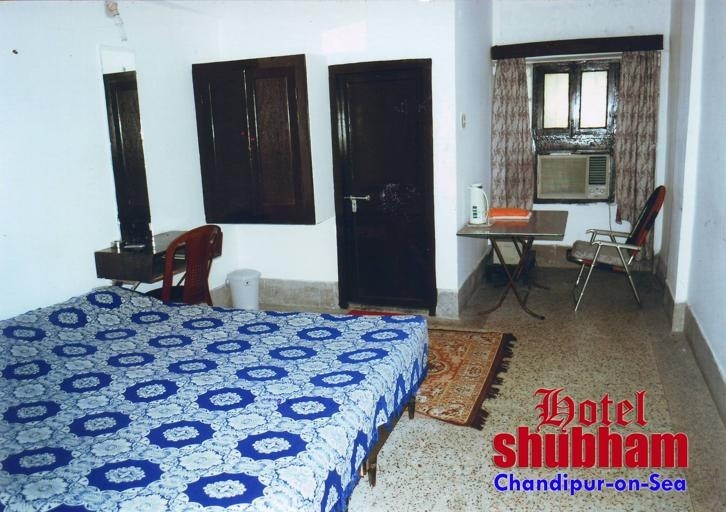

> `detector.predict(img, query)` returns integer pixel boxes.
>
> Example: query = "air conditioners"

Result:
[537,154,611,199]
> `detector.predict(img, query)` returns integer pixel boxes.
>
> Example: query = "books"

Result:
[162,252,187,260]
[490,206,533,220]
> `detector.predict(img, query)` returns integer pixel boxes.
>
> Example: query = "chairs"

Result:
[145,225,222,306]
[566,186,666,311]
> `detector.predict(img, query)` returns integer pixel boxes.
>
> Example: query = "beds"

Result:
[1,286,429,512]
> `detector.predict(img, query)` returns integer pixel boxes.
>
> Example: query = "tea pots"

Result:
[467,183,489,225]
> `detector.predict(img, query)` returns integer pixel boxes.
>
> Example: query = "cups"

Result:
[114,240,121,249]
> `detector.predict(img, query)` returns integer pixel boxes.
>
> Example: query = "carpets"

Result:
[406,329,517,431]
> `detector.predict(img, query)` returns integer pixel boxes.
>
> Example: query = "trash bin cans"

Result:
[225,269,262,310]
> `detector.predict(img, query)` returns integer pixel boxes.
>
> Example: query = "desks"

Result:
[457,211,568,320]
[95,230,222,290]
